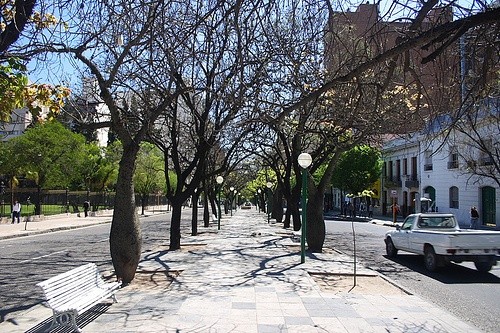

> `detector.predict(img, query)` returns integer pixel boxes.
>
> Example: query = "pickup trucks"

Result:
[384,213,500,272]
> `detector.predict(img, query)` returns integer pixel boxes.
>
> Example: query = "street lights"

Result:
[267,182,272,223]
[216,176,224,230]
[298,153,312,263]
[255,189,261,213]
[230,186,241,216]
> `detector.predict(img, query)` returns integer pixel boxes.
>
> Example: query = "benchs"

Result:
[35,263,122,333]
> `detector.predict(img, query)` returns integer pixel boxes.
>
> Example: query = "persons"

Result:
[391,203,399,218]
[82,200,89,217]
[470,207,479,226]
[343,201,352,216]
[368,204,373,217]
[11,200,21,224]
